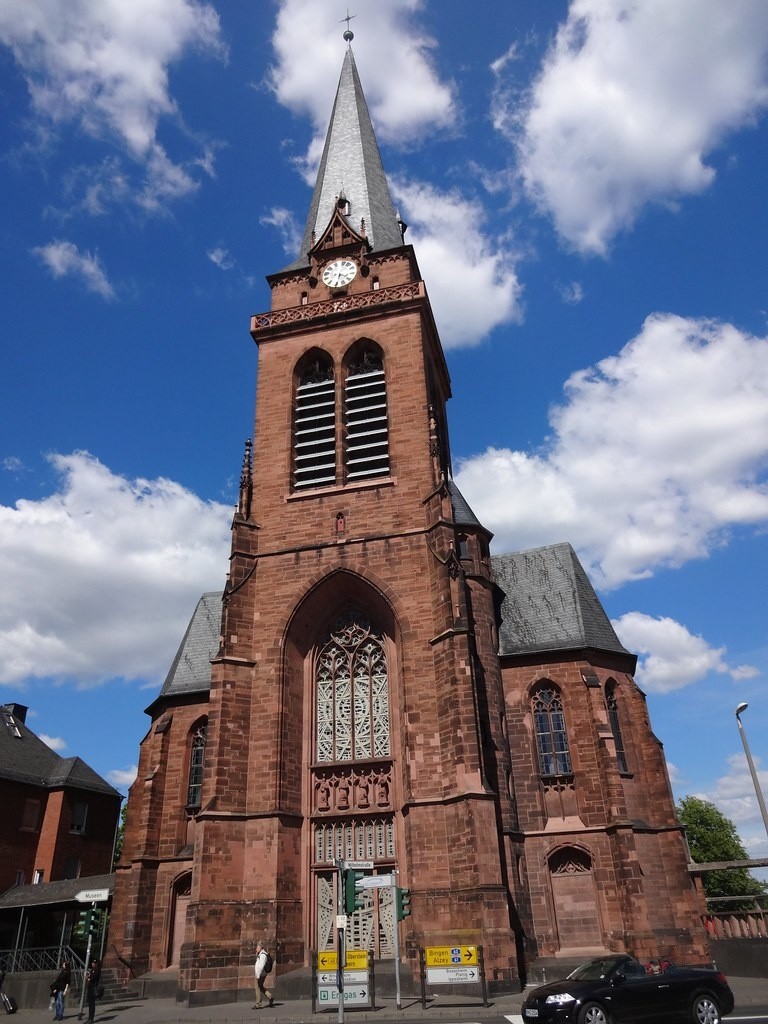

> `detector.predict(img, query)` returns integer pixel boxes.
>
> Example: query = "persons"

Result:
[53,962,72,1021]
[252,943,274,1009]
[84,959,99,1024]
[651,965,661,975]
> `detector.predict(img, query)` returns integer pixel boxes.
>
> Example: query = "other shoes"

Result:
[252,1002,262,1009]
[53,1016,62,1021]
[269,997,274,1007]
[83,1019,94,1024]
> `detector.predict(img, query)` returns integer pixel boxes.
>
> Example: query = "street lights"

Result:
[735,702,768,835]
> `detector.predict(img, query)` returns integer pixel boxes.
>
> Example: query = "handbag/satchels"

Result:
[94,985,104,998]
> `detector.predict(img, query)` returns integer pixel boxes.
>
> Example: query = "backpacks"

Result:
[260,952,273,973]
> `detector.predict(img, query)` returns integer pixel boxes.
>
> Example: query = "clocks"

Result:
[322,261,357,287]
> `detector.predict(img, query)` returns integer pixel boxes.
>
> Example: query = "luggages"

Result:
[1,992,18,1014]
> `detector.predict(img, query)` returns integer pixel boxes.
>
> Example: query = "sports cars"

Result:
[521,952,735,1024]
